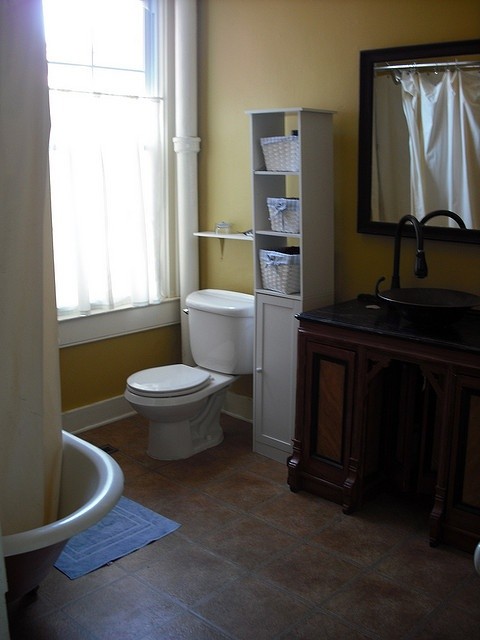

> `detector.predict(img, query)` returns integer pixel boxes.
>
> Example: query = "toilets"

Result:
[123,286,254,461]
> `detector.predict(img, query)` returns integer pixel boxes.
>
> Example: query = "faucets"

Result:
[420,210,467,230]
[386,214,431,285]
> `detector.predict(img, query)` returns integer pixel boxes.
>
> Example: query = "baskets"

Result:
[260,136,299,172]
[267,197,299,234]
[259,247,300,295]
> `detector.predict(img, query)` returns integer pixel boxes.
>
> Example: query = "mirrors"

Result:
[357,38,480,245]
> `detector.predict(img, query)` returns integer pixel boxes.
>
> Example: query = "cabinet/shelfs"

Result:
[285,330,366,516]
[244,108,339,465]
[428,367,480,554]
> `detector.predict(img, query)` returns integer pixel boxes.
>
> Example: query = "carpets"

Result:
[53,492,181,581]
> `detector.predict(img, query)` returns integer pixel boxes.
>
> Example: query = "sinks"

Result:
[378,286,477,307]
